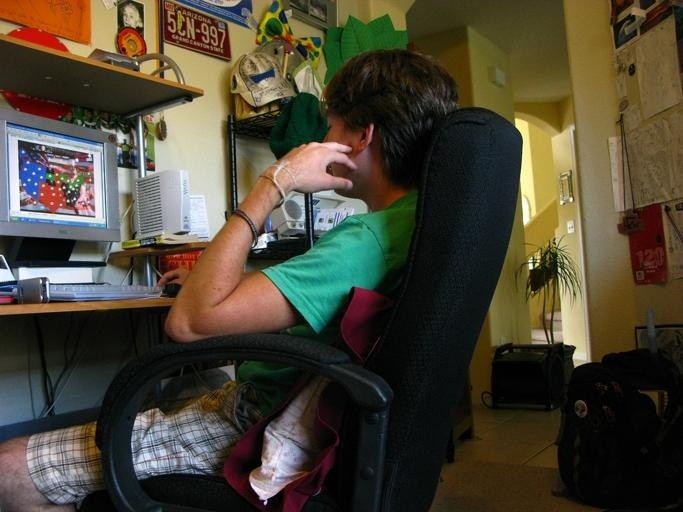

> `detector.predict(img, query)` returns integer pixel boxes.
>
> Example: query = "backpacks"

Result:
[550,347,683,512]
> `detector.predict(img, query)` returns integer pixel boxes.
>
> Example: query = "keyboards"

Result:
[44,283,161,301]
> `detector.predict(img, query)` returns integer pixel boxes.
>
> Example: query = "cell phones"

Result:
[15,277,50,304]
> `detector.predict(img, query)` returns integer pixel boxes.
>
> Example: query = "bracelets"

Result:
[233,210,258,249]
[256,172,285,212]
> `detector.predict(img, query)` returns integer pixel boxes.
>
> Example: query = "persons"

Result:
[0,45,460,512]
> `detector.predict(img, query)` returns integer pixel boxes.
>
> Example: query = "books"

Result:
[121,232,198,249]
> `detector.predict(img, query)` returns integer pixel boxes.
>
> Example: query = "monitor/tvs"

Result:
[0,106,122,243]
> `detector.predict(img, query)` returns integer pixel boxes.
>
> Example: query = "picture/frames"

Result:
[558,169,574,206]
[634,324,683,374]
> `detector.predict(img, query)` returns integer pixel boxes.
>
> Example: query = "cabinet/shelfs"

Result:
[229,111,371,258]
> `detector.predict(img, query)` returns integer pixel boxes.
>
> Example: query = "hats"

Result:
[229,52,297,108]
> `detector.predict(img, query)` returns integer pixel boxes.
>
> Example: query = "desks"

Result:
[0,298,176,319]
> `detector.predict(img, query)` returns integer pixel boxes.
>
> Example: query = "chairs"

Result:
[94,106,525,512]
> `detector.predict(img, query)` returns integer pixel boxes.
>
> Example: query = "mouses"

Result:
[164,282,181,297]
